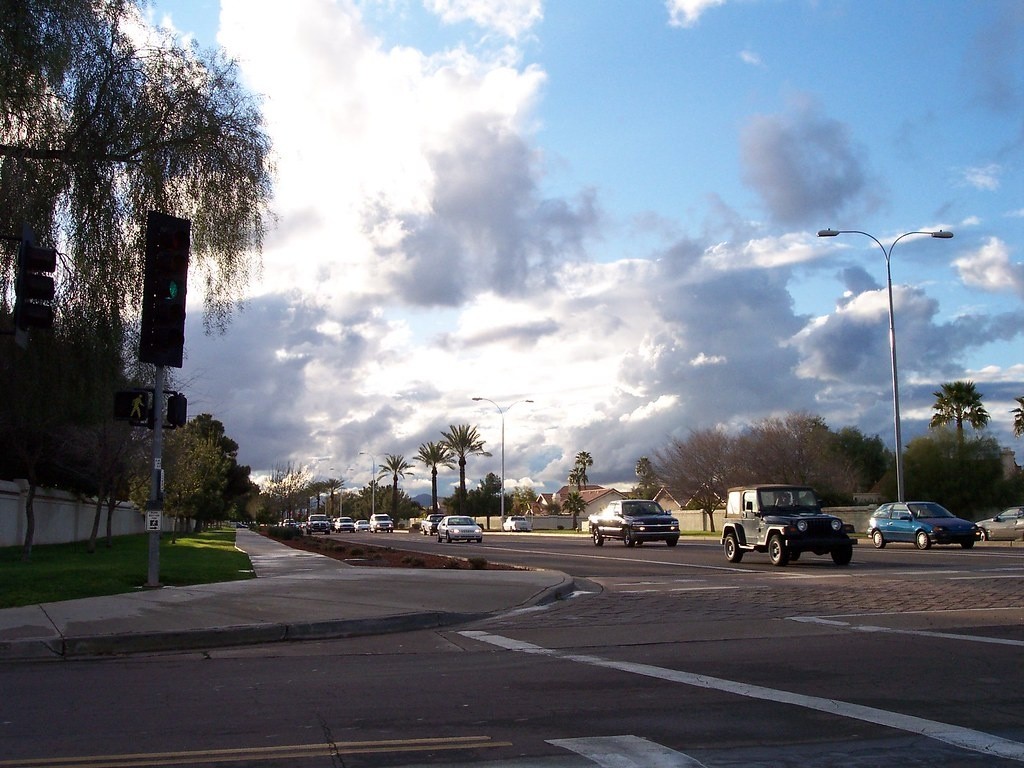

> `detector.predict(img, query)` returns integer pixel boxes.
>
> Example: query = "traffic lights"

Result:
[13,228,59,335]
[139,207,193,369]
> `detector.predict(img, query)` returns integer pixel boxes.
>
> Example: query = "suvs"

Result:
[306,514,330,535]
[370,513,394,533]
[719,483,859,569]
[335,517,355,533]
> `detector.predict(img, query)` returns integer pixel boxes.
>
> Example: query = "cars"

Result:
[973,505,1024,541]
[354,520,371,531]
[277,517,306,529]
[436,515,483,543]
[864,501,978,550]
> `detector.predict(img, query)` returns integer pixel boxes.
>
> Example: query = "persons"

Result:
[774,493,791,507]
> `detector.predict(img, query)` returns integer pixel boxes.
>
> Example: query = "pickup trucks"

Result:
[421,513,445,536]
[586,500,682,547]
[503,516,534,532]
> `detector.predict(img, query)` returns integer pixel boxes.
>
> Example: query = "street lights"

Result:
[329,467,354,517]
[815,228,954,502]
[358,451,391,511]
[472,397,536,515]
